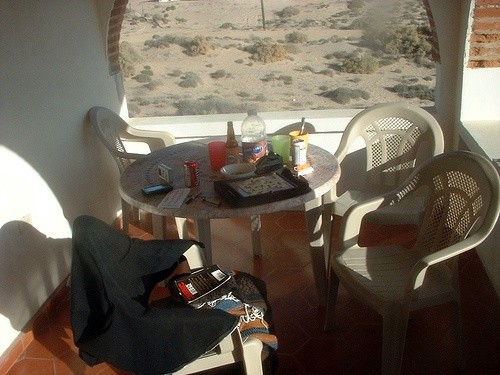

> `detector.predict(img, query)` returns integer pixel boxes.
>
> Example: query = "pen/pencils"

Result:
[186,190,202,204]
[299,117,305,134]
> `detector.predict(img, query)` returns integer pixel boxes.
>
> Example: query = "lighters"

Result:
[201,195,221,208]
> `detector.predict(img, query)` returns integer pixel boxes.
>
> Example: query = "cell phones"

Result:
[141,184,173,196]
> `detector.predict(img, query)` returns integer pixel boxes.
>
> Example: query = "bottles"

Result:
[240,105,267,162]
[227,120,240,164]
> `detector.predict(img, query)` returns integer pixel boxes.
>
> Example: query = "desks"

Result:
[103,129,337,297]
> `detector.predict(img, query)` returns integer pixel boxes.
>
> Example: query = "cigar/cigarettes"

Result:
[186,190,203,204]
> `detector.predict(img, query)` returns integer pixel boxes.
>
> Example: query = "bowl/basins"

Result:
[220,162,257,181]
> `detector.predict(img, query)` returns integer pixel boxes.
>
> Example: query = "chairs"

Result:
[88,104,195,242]
[318,103,447,292]
[324,150,500,375]
[70,216,271,375]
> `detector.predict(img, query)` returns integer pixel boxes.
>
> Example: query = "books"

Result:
[175,264,234,310]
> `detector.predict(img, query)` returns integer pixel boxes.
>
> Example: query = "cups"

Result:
[271,135,291,165]
[290,131,309,154]
[208,141,227,171]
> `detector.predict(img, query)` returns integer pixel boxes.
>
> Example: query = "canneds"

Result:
[183,160,200,187]
[292,139,306,167]
